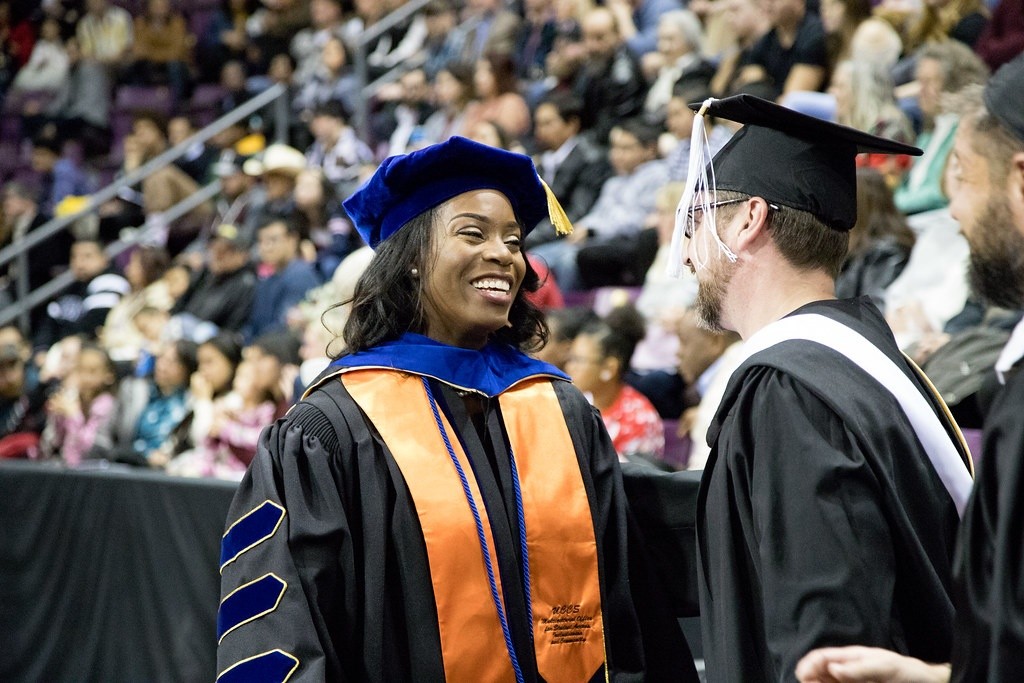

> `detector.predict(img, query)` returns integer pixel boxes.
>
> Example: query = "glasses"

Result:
[678,197,778,239]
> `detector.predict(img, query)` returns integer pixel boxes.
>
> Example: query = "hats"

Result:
[342,135,574,248]
[665,94,923,280]
[240,145,305,176]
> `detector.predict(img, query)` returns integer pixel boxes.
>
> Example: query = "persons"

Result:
[796,53,1024,683]
[1,1,1024,483]
[214,136,701,683]
[666,92,977,683]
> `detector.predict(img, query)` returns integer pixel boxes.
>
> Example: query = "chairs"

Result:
[0,9,233,172]
